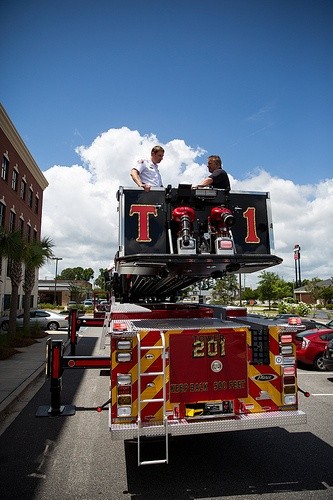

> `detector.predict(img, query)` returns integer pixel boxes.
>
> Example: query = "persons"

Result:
[196,155,231,190]
[130,145,165,193]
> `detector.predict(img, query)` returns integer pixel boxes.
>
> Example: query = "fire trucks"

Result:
[35,183,310,468]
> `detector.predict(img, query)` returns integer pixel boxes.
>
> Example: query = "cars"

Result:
[322,339,333,371]
[273,314,333,330]
[295,328,333,371]
[83,299,108,307]
[67,301,77,306]
[1,309,70,330]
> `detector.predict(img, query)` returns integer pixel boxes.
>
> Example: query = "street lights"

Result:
[51,257,62,305]
